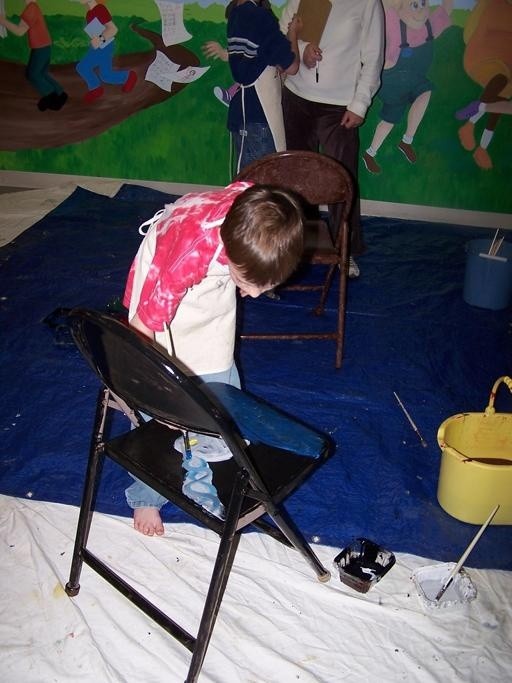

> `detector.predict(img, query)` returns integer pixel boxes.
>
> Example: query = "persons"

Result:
[278,1,385,278]
[459,0,511,169]
[203,42,242,106]
[124,180,302,537]
[0,0,66,110]
[77,0,136,101]
[361,0,454,174]
[456,100,511,120]
[225,1,302,180]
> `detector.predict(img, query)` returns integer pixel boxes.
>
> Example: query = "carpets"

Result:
[0,184,511,557]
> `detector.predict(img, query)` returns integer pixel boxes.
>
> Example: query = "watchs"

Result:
[99,34,108,45]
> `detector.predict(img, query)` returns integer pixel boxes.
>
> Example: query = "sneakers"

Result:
[337,255,360,279]
[472,146,494,170]
[213,84,233,107]
[37,90,59,112]
[396,140,417,164]
[49,90,69,111]
[458,122,478,151]
[83,86,105,102]
[453,99,482,121]
[122,70,138,93]
[361,152,381,174]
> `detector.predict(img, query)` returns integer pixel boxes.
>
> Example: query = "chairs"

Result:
[65,306,337,683]
[232,151,352,369]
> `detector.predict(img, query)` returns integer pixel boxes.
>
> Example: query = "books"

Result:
[294,1,333,45]
[160,66,211,84]
[155,0,193,46]
[146,50,180,93]
[1,1,8,39]
[85,18,117,49]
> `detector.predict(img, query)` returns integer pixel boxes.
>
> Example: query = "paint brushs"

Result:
[434,504,500,602]
[489,227,505,256]
[393,391,428,448]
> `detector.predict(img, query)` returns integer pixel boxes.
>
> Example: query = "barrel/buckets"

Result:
[462,238,512,310]
[437,377,512,525]
